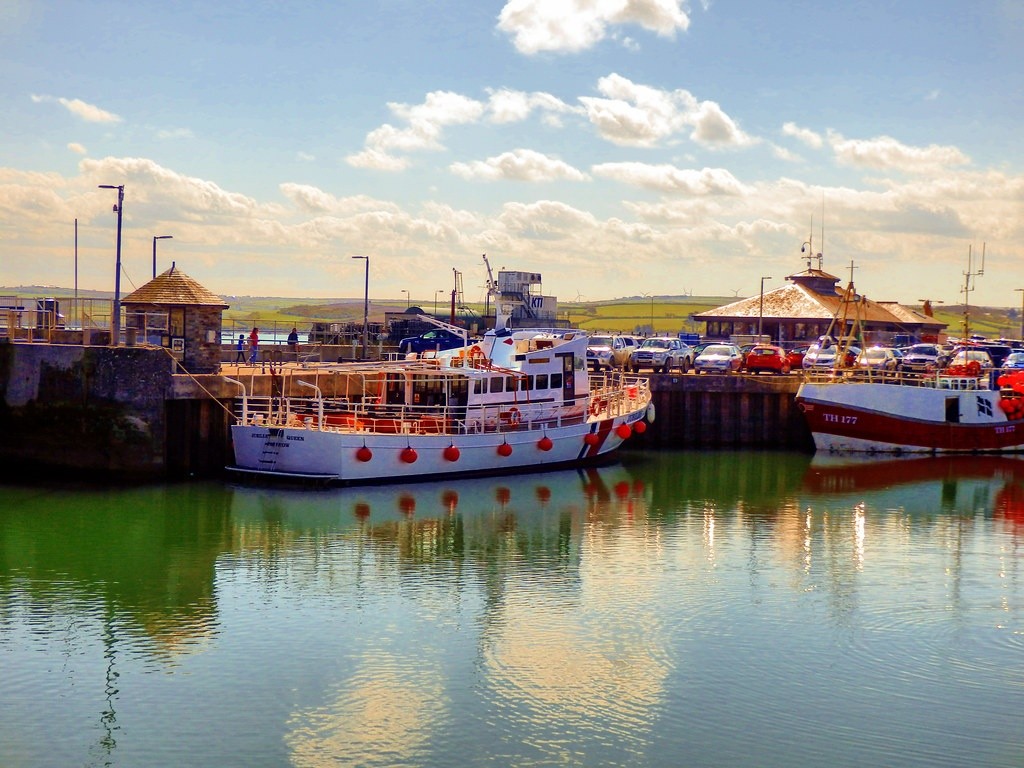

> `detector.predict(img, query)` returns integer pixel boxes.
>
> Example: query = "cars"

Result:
[398,330,476,352]
[696,335,1024,381]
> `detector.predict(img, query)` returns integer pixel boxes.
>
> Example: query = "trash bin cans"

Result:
[125,327,138,346]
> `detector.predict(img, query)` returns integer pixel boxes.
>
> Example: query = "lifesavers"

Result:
[471,345,481,358]
[592,398,602,417]
[508,408,521,430]
[967,362,980,377]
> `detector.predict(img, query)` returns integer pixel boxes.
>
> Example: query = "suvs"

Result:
[587,333,699,373]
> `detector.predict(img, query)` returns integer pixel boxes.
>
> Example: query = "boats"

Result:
[795,366,1024,454]
[230,255,652,482]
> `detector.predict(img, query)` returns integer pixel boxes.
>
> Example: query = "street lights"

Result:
[648,296,658,329]
[98,184,126,346]
[152,235,176,278]
[1013,289,1024,341]
[759,277,772,343]
[801,214,818,274]
[401,290,409,308]
[435,290,444,315]
[353,256,370,357]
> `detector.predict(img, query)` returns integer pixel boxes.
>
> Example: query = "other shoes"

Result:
[298,354,301,356]
[253,365,257,366]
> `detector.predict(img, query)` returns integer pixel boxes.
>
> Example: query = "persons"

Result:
[236,335,248,366]
[287,328,298,345]
[246,328,259,366]
